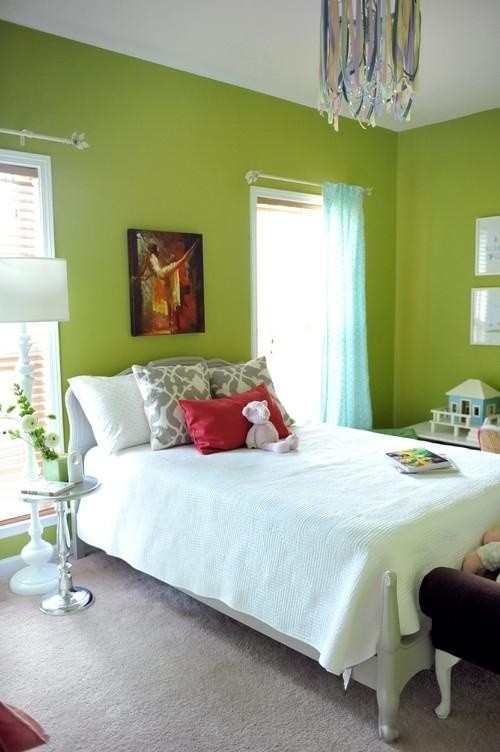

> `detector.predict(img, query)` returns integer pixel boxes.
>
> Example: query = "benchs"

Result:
[419,529,500,717]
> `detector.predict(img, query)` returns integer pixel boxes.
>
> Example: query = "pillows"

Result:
[67,374,151,453]
[209,356,295,427]
[132,362,212,450]
[178,382,291,455]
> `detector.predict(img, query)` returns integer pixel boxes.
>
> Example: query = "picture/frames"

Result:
[467,286,500,346]
[472,216,500,276]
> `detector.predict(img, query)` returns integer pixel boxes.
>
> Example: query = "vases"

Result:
[44,456,68,481]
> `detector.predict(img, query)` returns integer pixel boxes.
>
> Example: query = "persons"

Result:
[130,238,200,335]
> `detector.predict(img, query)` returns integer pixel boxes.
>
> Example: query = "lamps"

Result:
[315,0,423,128]
[0,255,70,593]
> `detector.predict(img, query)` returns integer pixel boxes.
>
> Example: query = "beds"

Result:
[64,354,499,744]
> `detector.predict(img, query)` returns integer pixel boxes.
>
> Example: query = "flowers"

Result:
[0,382,58,460]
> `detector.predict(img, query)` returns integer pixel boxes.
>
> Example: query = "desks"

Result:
[22,476,101,615]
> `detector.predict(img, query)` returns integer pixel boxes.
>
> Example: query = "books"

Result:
[395,454,460,475]
[21,480,75,496]
[385,446,449,471]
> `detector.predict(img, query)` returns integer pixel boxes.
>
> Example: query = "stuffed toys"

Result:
[461,529,500,582]
[242,400,299,454]
